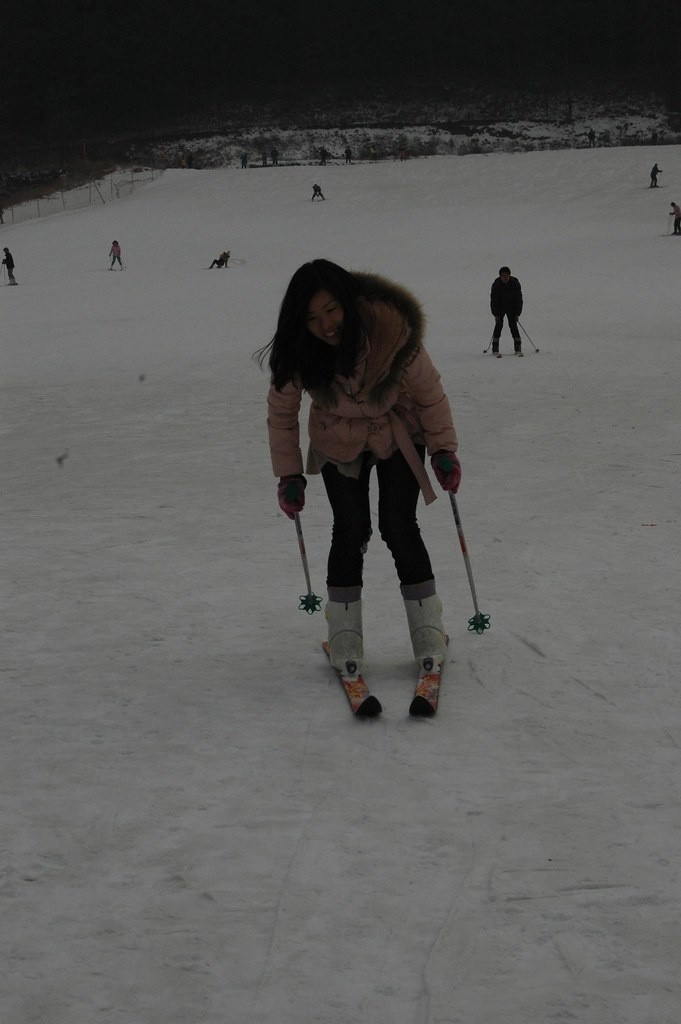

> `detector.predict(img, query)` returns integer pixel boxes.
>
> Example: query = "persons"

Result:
[271,147,278,167]
[398,145,407,162]
[253,260,463,668]
[0,207,3,224]
[262,151,268,167]
[490,267,524,355]
[588,129,595,148]
[651,131,657,145]
[371,145,377,162]
[210,250,231,268]
[651,163,663,187]
[669,202,681,234]
[2,247,16,284]
[240,152,247,169]
[344,147,351,164]
[312,184,324,200]
[109,241,122,270]
[319,147,328,165]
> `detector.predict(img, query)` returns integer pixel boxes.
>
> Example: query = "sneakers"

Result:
[325,584,365,671]
[398,574,448,667]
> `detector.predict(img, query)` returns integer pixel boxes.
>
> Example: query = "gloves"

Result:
[431,450,461,495]
[277,474,307,520]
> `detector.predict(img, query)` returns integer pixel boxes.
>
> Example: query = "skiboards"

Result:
[107,264,125,271]
[663,231,681,236]
[321,586,451,719]
[0,281,25,287]
[645,184,670,189]
[202,264,230,269]
[492,344,524,358]
